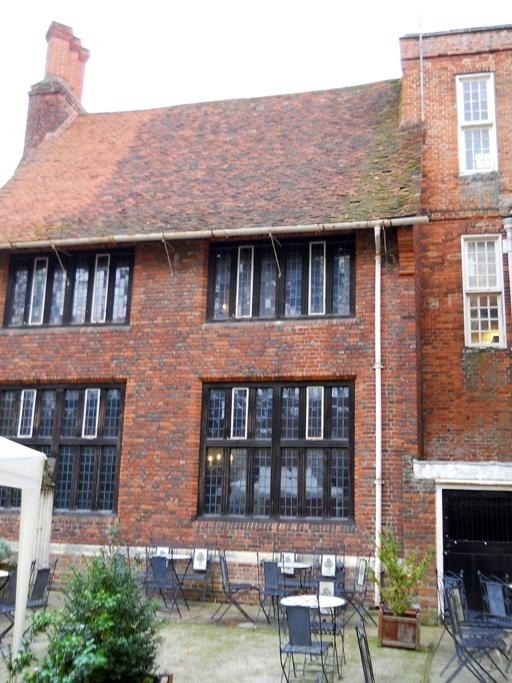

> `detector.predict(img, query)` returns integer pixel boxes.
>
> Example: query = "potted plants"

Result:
[374,527,435,651]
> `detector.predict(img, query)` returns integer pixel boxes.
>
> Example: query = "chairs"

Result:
[355,625,377,683]
[278,576,346,683]
[433,568,512,682]
[209,549,256,624]
[256,545,378,631]
[141,543,218,620]
